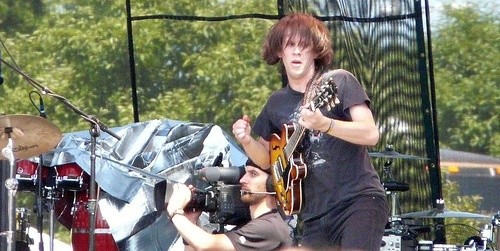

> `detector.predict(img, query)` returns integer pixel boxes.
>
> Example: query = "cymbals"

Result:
[0,114,62,161]
[367,149,431,160]
[392,209,496,219]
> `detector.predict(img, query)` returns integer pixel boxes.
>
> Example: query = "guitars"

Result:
[267,76,342,220]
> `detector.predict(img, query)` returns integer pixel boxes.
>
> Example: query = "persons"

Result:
[231,13,389,251]
[167,156,291,251]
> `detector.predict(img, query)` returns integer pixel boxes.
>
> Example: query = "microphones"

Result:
[39,97,47,119]
[206,167,220,181]
[244,190,276,195]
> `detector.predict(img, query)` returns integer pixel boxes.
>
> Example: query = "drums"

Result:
[55,160,89,194]
[15,154,52,189]
[70,189,120,251]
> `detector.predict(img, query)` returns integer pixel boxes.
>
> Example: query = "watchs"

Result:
[168,209,186,223]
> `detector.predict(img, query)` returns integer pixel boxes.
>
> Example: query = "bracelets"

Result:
[322,119,335,134]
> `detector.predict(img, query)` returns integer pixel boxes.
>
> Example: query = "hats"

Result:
[246,157,272,175]
